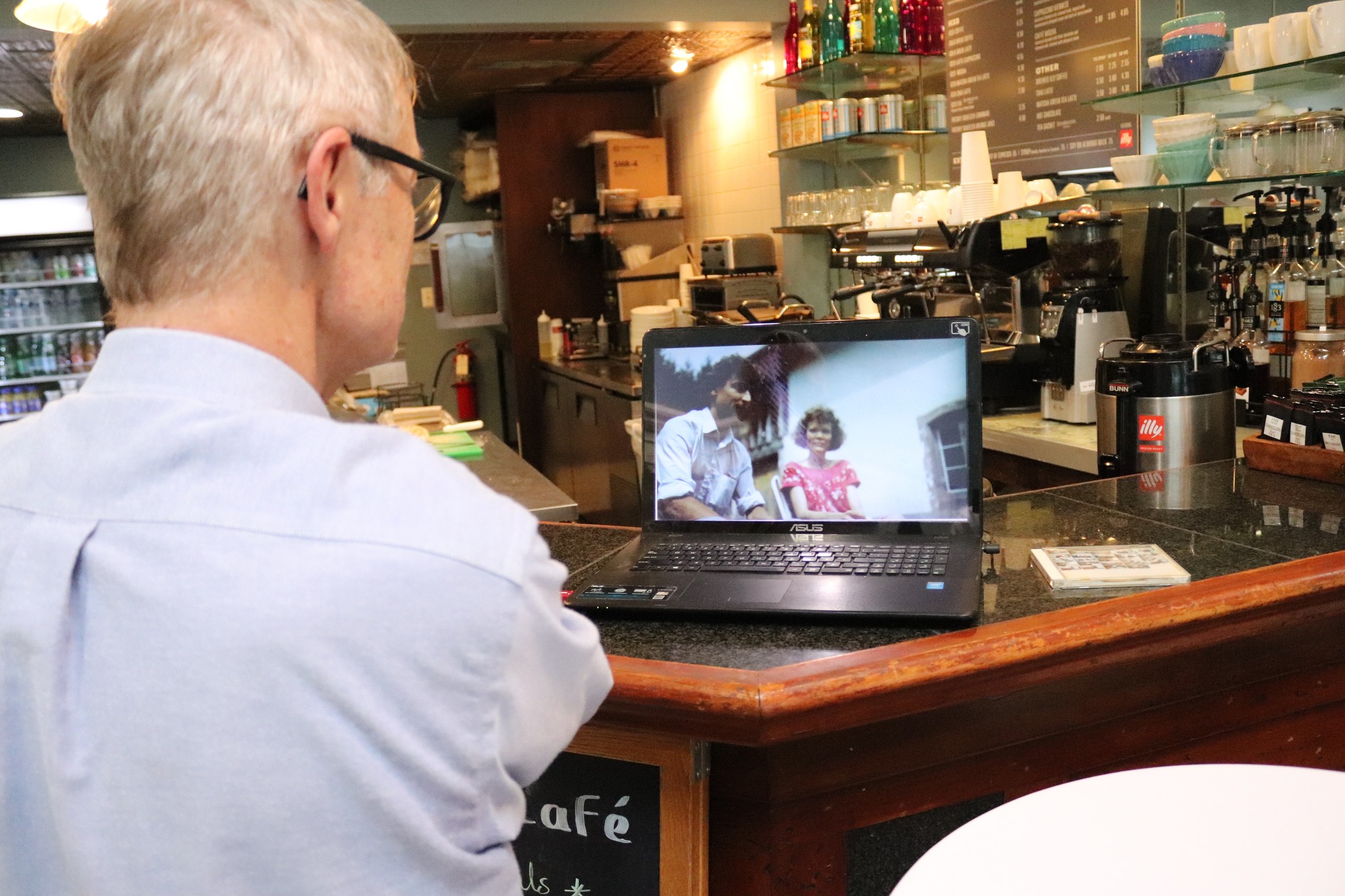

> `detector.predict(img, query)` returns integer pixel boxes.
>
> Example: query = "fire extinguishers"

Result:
[430,340,478,421]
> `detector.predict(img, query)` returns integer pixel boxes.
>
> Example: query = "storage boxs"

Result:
[595,138,669,211]
[344,360,408,391]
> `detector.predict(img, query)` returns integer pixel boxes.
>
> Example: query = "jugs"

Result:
[598,187,640,218]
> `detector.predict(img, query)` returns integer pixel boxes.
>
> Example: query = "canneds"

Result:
[820,94,905,140]
[904,94,946,132]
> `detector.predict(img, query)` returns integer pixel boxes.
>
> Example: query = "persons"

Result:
[657,355,775,521]
[781,407,869,521]
[0,0,614,896]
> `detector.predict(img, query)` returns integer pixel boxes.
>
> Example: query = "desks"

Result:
[450,431,579,522]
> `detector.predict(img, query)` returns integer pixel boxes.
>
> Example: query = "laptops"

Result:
[559,318,983,621]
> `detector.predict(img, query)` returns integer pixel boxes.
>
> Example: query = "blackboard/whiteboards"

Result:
[513,729,712,896]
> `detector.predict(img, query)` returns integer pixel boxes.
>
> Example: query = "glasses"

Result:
[296,132,457,241]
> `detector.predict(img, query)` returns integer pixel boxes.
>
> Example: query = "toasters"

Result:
[700,232,778,280]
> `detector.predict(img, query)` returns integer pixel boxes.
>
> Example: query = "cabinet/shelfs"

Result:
[765,51,945,234]
[1078,51,1345,203]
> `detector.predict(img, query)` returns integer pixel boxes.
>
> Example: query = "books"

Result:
[1030,544,1191,589]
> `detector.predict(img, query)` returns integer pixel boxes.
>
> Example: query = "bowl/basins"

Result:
[620,245,653,271]
[1147,11,1234,91]
[629,305,675,353]
[1110,112,1221,187]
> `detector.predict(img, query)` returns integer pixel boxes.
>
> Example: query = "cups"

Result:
[1268,11,1310,65]
[1208,121,1260,179]
[787,129,1118,230]
[1294,110,1345,174]
[1233,22,1274,73]
[1250,115,1295,175]
[1306,0,1345,58]
[667,263,694,314]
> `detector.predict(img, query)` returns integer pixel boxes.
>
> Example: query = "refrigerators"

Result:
[0,188,115,426]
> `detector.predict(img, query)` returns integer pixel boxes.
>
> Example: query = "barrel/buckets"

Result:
[1092,330,1237,478]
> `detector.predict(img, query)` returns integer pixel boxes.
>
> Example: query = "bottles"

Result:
[1198,210,1345,422]
[784,0,945,87]
[536,290,618,364]
[0,246,106,416]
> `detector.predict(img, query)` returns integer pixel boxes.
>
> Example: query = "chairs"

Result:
[771,475,793,520]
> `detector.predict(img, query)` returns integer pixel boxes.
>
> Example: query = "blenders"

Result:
[1040,215,1134,426]
